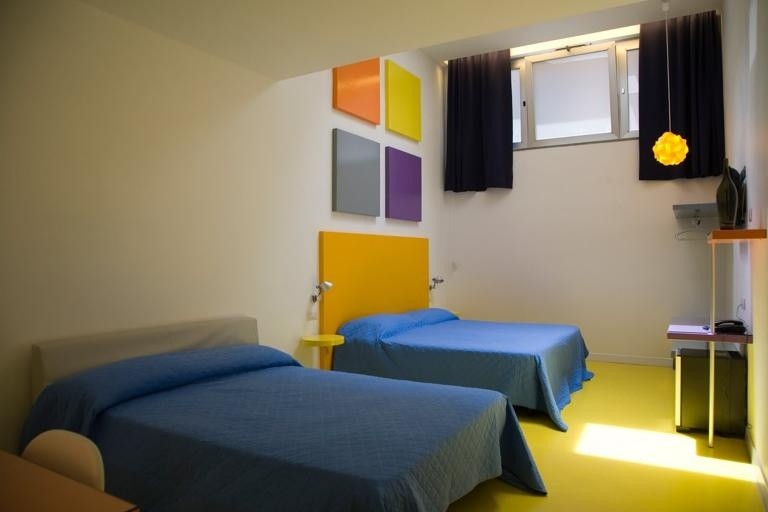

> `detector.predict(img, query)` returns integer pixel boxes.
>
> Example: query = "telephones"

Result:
[715,320,746,334]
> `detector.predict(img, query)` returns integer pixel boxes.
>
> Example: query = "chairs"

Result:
[19,427,108,493]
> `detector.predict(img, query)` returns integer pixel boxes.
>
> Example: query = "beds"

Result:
[34,314,548,512]
[319,231,595,432]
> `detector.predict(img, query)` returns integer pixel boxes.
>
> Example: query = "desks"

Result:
[2,448,138,511]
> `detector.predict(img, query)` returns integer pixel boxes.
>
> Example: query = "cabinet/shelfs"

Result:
[665,228,766,449]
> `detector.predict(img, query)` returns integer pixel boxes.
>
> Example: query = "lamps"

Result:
[429,276,444,290]
[312,280,333,303]
